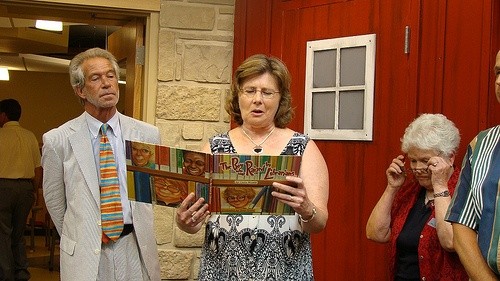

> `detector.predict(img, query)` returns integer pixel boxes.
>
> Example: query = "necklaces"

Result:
[240,127,276,154]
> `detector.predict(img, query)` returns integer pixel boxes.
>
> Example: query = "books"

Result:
[124,139,302,215]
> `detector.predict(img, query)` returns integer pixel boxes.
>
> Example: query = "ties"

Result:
[99,124,124,243]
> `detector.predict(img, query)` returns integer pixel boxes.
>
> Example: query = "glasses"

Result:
[400,152,442,174]
[239,88,280,98]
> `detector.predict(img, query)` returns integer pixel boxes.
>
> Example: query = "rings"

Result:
[434,161,440,166]
[192,218,196,222]
[291,196,294,201]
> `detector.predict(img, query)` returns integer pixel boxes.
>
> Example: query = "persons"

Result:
[366,113,469,281]
[41,48,160,281]
[445,49,500,281]
[0,99,43,281]
[175,55,329,281]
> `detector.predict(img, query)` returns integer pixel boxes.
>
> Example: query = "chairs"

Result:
[27,192,61,273]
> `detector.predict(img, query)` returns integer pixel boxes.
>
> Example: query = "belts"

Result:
[120,224,133,237]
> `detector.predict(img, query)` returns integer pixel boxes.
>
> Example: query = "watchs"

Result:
[433,190,451,198]
[299,205,317,222]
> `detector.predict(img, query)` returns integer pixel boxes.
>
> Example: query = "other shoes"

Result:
[15,269,30,281]
